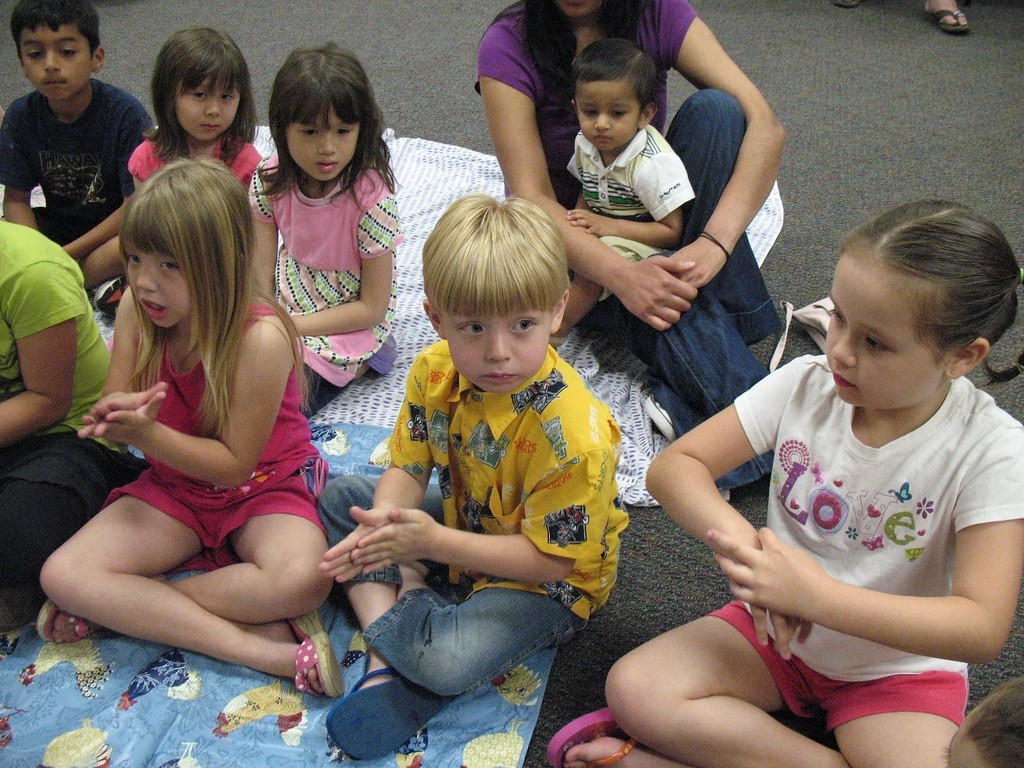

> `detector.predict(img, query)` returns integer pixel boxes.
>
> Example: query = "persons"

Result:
[943,677,1024,768]
[317,196,627,761]
[543,38,696,346]
[250,43,401,418]
[474,0,785,491]
[546,199,1024,767]
[0,0,155,320]
[36,157,345,698]
[0,219,131,635]
[833,0,970,32]
[129,28,263,201]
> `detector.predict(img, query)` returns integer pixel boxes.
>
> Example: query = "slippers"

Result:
[834,0,861,7]
[922,3,971,32]
[547,707,639,768]
[326,668,465,760]
[287,607,345,697]
[36,598,94,643]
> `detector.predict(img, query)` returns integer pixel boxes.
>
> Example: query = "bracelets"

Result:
[701,231,730,260]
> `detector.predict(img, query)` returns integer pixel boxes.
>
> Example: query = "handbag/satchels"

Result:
[780,296,835,355]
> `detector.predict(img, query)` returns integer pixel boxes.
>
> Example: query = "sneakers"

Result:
[94,276,128,317]
[645,394,676,444]
